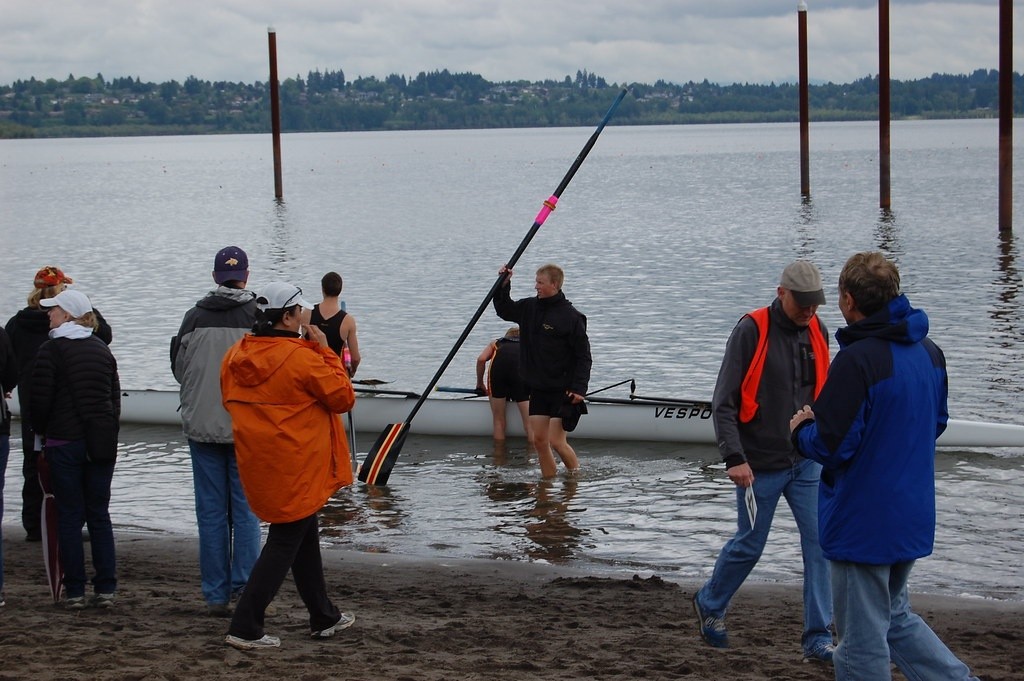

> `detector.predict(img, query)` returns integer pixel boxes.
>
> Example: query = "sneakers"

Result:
[693,593,729,648]
[66,597,84,611]
[88,594,114,607]
[225,634,281,649]
[808,645,834,665]
[311,612,355,639]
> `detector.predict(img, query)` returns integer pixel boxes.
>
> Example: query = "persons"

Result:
[169,245,260,608]
[493,263,592,476]
[692,260,836,662]
[788,250,981,681]
[27,289,120,610]
[0,325,17,606]
[0,266,111,543]
[220,282,356,648]
[301,272,361,379]
[476,327,534,443]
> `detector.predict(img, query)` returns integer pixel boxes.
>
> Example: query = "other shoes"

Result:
[208,605,235,620]
[25,531,40,541]
[83,530,90,541]
[231,593,244,603]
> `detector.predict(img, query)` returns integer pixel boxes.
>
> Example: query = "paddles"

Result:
[341,301,362,475]
[435,386,711,407]
[353,388,415,395]
[357,82,638,486]
[351,379,398,385]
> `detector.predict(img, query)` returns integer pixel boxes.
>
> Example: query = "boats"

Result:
[5,382,1024,447]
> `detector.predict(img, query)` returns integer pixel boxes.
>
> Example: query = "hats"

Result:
[214,246,248,284]
[34,266,72,289]
[40,290,93,318]
[256,281,314,312]
[780,260,825,307]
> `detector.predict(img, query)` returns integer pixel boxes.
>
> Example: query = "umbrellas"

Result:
[37,440,62,603]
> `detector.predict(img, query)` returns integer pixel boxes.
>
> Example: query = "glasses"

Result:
[281,287,303,311]
